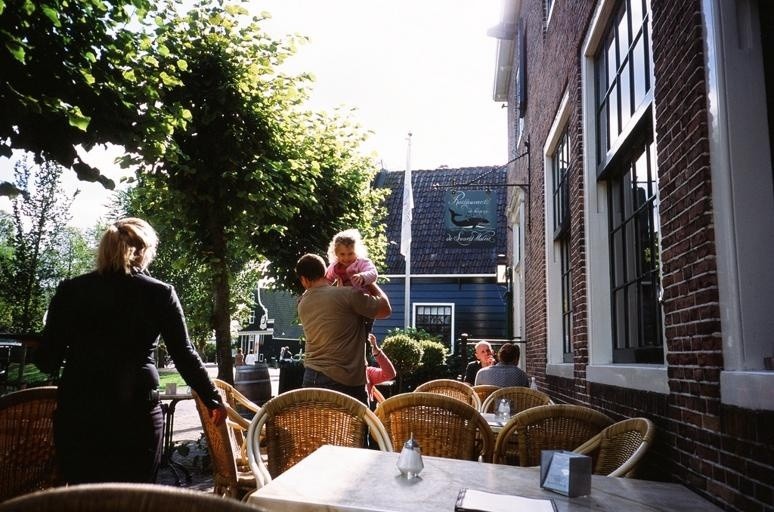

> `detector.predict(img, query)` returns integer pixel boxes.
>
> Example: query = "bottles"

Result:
[396,431,424,482]
[530,376,537,390]
[494,398,511,422]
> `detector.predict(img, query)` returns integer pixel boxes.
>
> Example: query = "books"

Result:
[455,487,559,512]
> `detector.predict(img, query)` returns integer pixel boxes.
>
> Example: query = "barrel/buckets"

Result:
[235,361,272,419]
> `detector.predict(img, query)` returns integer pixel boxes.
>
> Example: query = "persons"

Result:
[474,343,531,389]
[283,346,294,367]
[279,347,285,367]
[302,235,379,366]
[29,216,228,489]
[234,349,245,368]
[464,341,499,386]
[364,332,397,412]
[295,253,393,406]
[245,348,258,366]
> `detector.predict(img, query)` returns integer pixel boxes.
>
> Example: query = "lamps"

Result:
[495,253,511,286]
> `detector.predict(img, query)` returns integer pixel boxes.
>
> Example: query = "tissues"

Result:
[165,383,177,395]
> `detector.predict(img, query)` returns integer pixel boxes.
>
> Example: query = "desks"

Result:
[250,444,726,512]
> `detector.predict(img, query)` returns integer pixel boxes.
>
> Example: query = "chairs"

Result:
[0,376,662,511]
